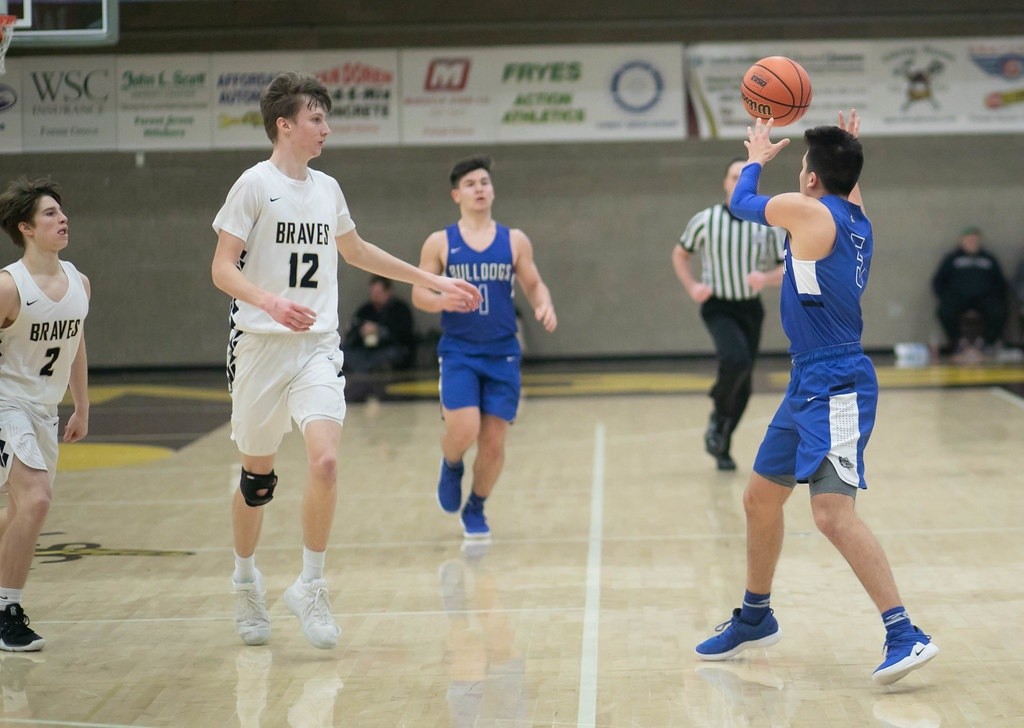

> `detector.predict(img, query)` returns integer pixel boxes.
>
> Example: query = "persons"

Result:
[929,225,1011,365]
[211,72,484,649]
[0,173,90,652]
[695,108,938,686]
[670,156,789,474]
[346,274,416,417]
[413,154,560,541]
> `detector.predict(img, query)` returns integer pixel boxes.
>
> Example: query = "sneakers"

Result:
[435,457,465,514]
[229,569,271,646]
[871,625,940,686]
[459,500,491,538]
[0,603,45,652]
[695,607,782,661]
[283,572,341,650]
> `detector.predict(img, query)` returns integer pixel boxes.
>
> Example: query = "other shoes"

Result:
[705,414,734,455]
[714,451,736,471]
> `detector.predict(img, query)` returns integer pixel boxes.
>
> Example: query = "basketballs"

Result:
[741,55,813,128]
